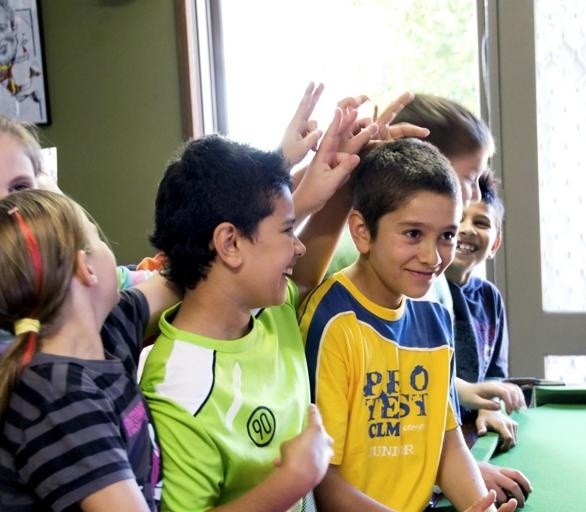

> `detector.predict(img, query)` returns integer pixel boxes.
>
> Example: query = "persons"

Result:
[138,91,431,510]
[0,0,17,67]
[0,109,360,511]
[443,165,520,453]
[0,93,382,358]
[297,138,520,511]
[386,93,535,505]
[125,81,326,273]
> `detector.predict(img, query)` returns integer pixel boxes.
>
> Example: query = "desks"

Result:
[435,385,586,512]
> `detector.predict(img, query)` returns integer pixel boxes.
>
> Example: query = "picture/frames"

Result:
[0,0,52,128]
